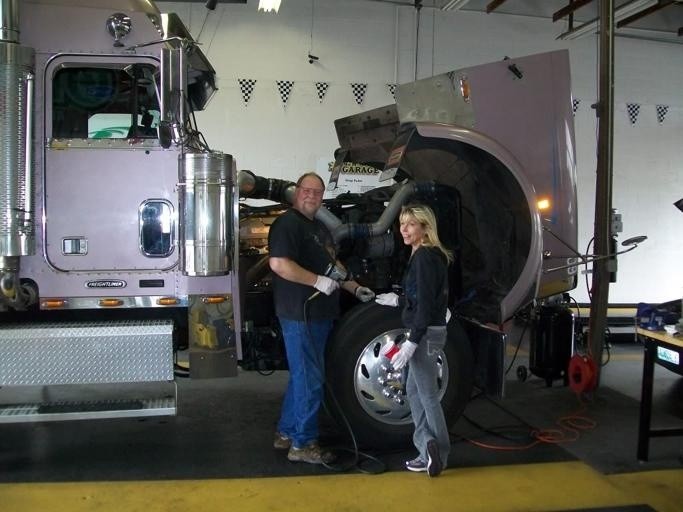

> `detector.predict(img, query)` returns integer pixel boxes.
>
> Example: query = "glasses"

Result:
[298,186,323,195]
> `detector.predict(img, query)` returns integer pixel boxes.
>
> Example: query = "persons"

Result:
[375,205,451,478]
[268,173,375,464]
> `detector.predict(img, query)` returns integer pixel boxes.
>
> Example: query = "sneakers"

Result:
[405,439,443,478]
[273,431,337,464]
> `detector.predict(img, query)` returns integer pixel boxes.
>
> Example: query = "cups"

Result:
[381,341,400,359]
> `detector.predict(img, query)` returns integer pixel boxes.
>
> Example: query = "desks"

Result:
[636,326,683,463]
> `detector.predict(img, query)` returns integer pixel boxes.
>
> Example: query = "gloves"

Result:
[390,340,418,371]
[355,286,399,307]
[313,275,340,296]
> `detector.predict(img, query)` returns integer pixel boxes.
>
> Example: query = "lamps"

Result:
[257,0,281,13]
[442,0,470,12]
[555,0,659,40]
[204,0,217,10]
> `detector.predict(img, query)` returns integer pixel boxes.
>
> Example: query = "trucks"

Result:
[0,2,646,447]
